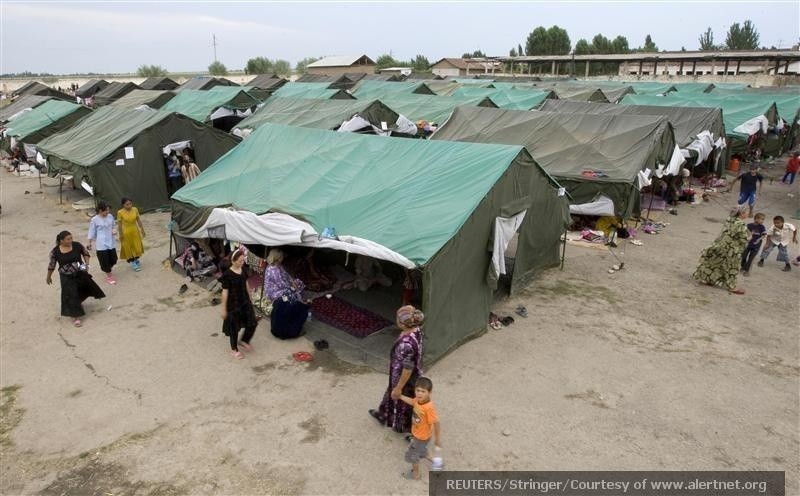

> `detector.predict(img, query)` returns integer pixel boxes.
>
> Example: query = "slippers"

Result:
[106,260,143,284]
[400,470,422,482]
[490,306,527,330]
[74,320,82,326]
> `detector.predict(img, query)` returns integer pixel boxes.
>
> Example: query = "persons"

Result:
[370,305,426,434]
[391,377,444,481]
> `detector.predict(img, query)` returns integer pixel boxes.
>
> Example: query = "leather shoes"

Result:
[178,284,189,292]
[211,297,221,307]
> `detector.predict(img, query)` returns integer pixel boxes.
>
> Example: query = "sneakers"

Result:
[739,259,791,277]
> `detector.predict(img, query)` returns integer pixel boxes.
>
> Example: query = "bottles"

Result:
[431,446,446,473]
[307,313,312,322]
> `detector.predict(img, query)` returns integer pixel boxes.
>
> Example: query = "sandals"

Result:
[368,408,388,426]
[229,339,254,361]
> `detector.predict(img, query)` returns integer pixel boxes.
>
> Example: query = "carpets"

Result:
[304,295,395,340]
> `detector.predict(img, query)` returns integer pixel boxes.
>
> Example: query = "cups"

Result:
[325,294,332,300]
